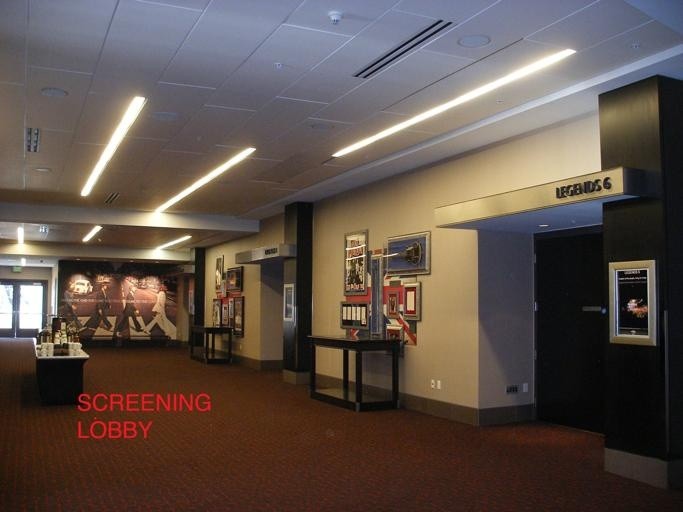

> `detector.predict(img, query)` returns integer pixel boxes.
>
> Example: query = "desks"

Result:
[189,326,234,365]
[305,333,401,413]
[31,337,91,407]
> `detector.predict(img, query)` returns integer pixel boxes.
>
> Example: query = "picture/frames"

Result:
[607,258,658,346]
[282,283,294,322]
[339,229,432,358]
[212,254,245,338]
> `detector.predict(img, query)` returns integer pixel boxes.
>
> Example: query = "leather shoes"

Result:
[136,328,143,332]
[143,329,151,335]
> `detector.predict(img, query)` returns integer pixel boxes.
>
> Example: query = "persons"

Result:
[87,283,115,331]
[142,283,173,337]
[60,279,87,334]
[116,285,143,332]
